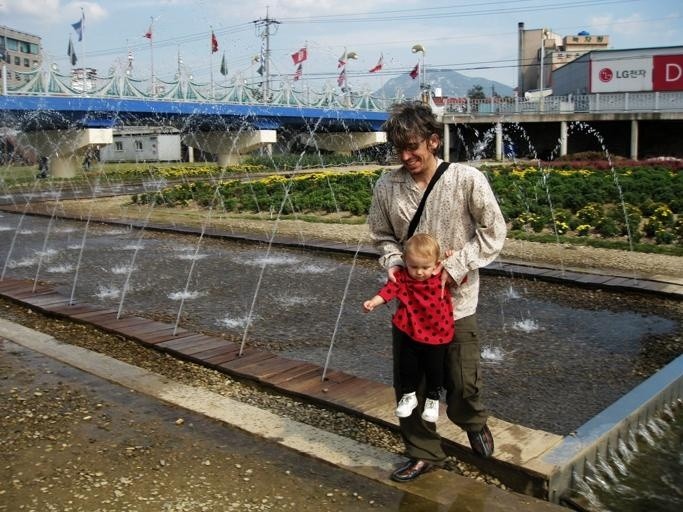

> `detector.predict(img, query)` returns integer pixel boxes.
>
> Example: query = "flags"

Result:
[67,40,78,65]
[409,63,419,79]
[211,30,219,52]
[71,13,84,42]
[294,64,302,80]
[220,52,228,75]
[337,69,345,87]
[291,48,307,65]
[369,63,384,73]
[336,58,347,68]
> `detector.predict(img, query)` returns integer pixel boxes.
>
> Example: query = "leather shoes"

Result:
[392,459,432,482]
[467,423,494,458]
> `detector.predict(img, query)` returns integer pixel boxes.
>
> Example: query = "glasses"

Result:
[391,138,426,155]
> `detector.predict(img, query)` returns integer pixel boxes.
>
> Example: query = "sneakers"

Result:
[421,398,439,422]
[395,391,418,418]
